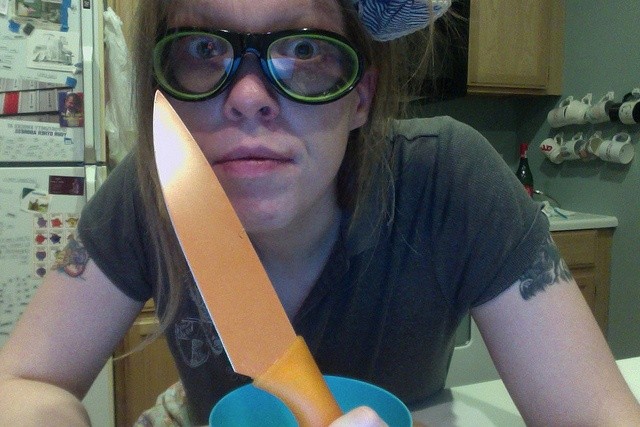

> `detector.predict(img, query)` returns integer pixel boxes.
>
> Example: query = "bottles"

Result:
[515,143,534,200]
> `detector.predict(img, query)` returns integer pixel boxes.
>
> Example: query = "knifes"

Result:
[151,88,345,427]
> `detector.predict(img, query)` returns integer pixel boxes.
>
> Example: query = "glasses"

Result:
[150,26,369,105]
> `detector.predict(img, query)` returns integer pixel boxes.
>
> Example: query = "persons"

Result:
[0,0,640,427]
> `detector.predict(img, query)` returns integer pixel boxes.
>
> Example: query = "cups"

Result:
[579,130,599,160]
[539,134,564,164]
[565,131,582,162]
[589,90,615,125]
[545,95,569,130]
[592,131,635,166]
[209,373,413,427]
[567,90,592,126]
[617,89,639,128]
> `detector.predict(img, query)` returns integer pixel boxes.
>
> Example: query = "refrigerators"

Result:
[2,1,116,427]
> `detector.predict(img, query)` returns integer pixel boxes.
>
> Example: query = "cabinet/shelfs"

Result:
[549,228,616,344]
[467,1,564,98]
[108,0,183,426]
